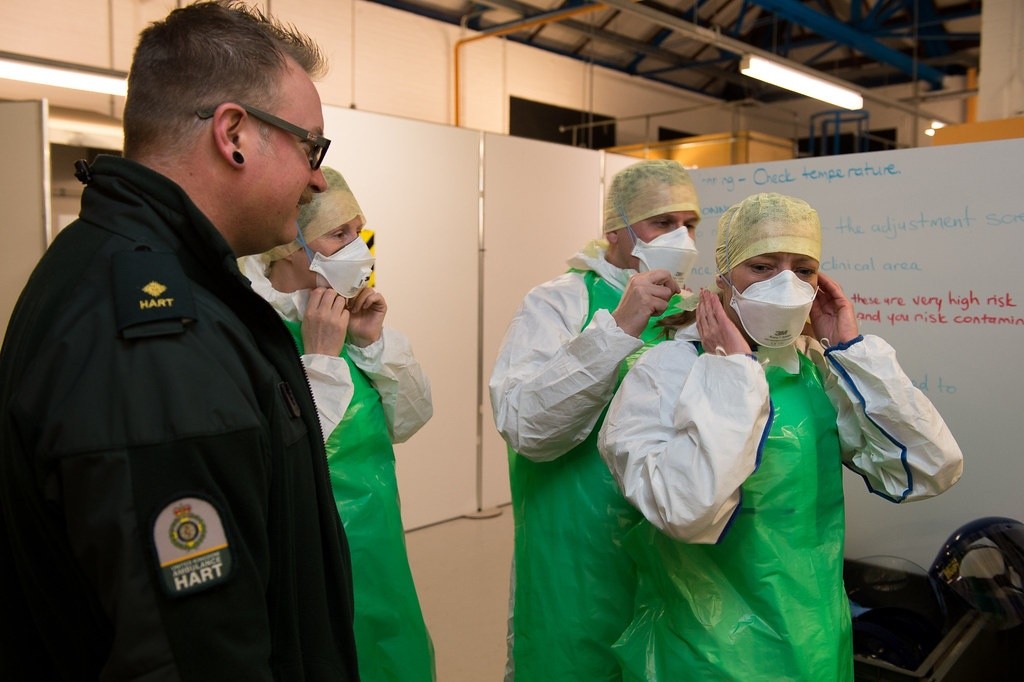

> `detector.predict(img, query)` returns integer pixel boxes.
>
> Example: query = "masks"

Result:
[618,212,698,288]
[722,270,819,349]
[295,223,374,298]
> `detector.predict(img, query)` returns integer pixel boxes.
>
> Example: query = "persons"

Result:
[595,190,965,682]
[235,163,440,682]
[487,159,702,682]
[0,0,363,682]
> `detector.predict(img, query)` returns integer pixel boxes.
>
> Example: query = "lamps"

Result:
[739,57,865,112]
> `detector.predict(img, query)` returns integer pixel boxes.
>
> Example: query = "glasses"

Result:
[196,102,331,171]
